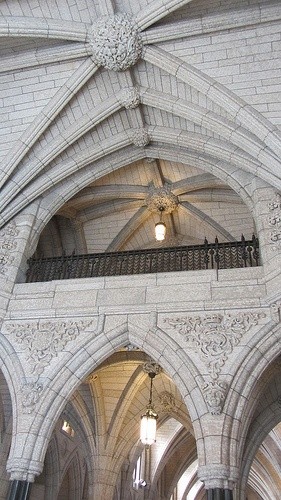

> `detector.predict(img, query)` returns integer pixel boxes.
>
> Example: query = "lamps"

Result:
[138,372,159,444]
[153,207,167,241]
